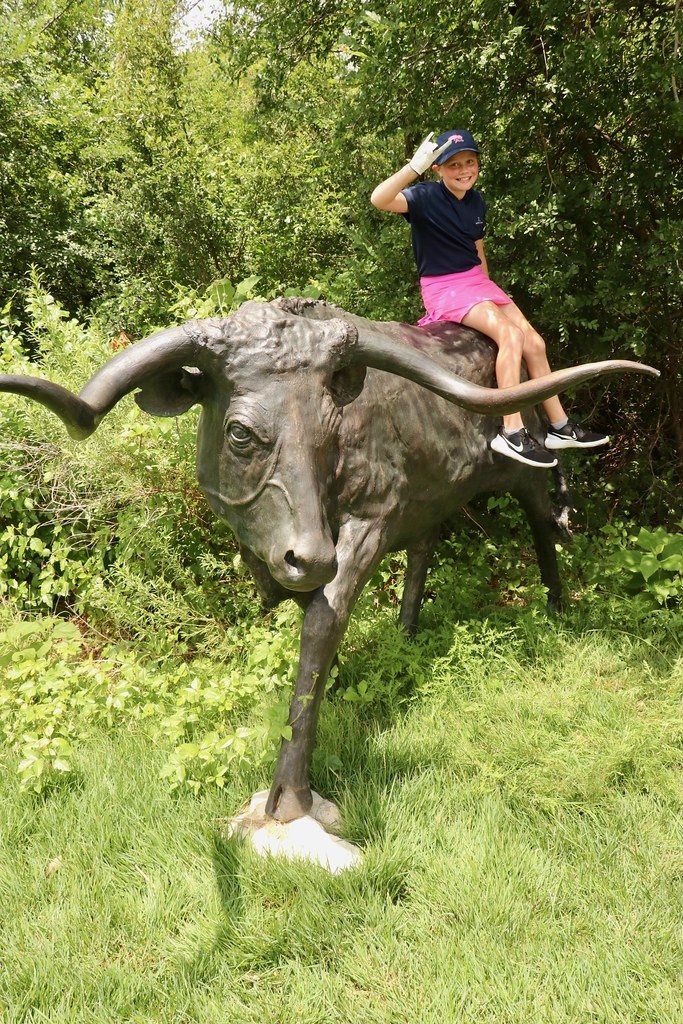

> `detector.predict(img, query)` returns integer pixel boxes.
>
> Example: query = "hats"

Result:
[432,130,481,166]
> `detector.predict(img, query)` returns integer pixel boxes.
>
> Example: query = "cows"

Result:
[0,292,661,824]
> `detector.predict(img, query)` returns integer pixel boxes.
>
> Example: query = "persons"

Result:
[370,129,610,467]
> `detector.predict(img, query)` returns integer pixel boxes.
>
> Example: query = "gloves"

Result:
[410,133,452,176]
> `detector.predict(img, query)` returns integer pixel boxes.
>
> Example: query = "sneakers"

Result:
[544,418,610,450]
[491,426,558,467]
